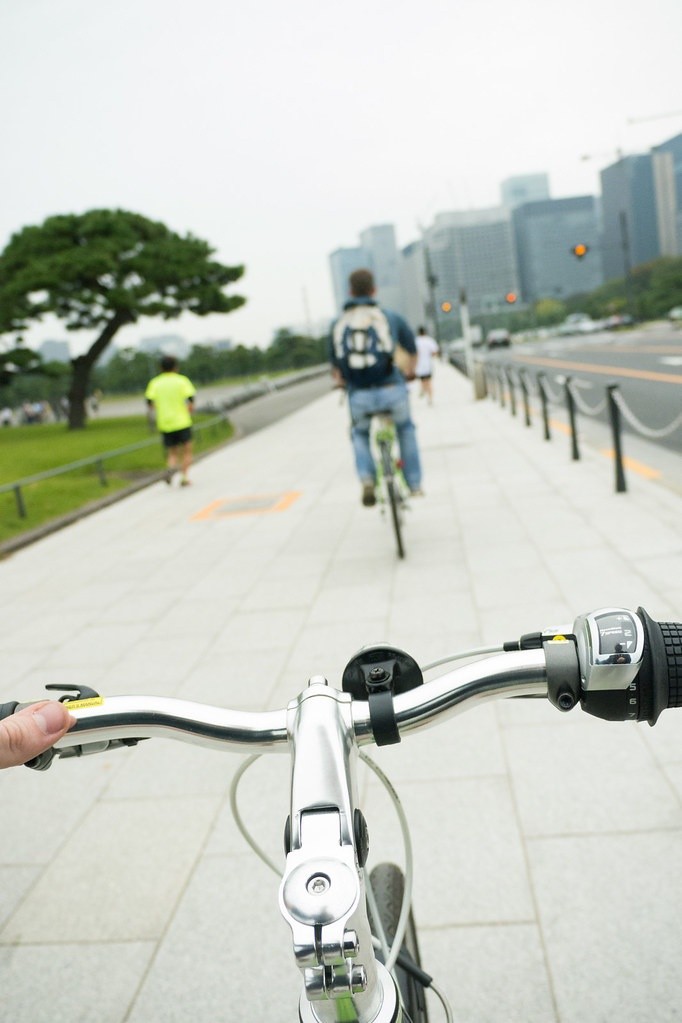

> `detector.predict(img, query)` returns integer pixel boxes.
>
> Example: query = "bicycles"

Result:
[0,609,682,1023]
[334,373,425,556]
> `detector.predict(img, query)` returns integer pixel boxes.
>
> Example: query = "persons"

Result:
[414,326,439,400]
[1,396,99,424]
[1,701,69,769]
[330,272,424,505]
[145,358,198,487]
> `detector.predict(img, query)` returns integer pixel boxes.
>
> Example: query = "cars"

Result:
[486,327,511,351]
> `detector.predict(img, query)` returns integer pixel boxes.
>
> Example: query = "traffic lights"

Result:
[575,245,589,259]
[507,292,515,304]
[442,301,451,313]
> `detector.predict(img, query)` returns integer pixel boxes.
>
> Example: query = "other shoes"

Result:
[363,486,376,508]
